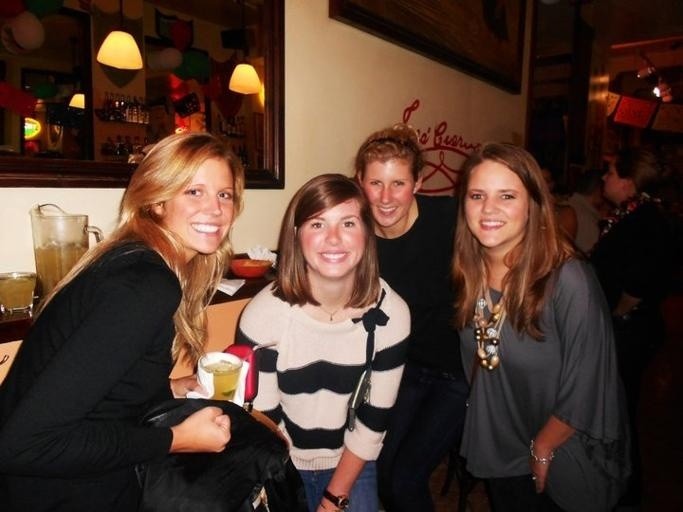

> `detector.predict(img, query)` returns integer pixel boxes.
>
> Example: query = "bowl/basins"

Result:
[231,259,272,278]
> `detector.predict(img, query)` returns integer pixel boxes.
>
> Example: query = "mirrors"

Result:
[0,0,95,161]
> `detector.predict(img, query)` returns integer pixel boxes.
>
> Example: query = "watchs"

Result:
[322,489,352,510]
[527,440,556,466]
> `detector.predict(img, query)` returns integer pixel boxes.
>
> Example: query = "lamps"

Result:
[69,93,85,110]
[653,81,674,105]
[96,0,144,71]
[228,0,261,97]
[636,61,657,82]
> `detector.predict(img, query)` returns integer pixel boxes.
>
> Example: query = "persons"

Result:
[234,172,411,512]
[0,128,233,512]
[449,139,636,512]
[354,128,460,512]
[538,134,673,418]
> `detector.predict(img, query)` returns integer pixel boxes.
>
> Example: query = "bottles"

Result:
[216,114,247,136]
[108,135,148,159]
[232,143,249,168]
[104,92,148,124]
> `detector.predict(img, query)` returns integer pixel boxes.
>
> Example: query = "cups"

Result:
[0,272,38,313]
[199,352,242,404]
[31,213,104,303]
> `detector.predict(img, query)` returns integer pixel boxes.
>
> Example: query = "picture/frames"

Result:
[328,1,527,94]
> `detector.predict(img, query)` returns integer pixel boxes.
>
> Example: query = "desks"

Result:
[1,248,278,387]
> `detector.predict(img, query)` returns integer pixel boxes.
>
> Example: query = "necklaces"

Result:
[471,265,517,371]
[307,294,352,325]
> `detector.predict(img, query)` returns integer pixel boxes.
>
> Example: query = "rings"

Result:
[531,474,537,482]
